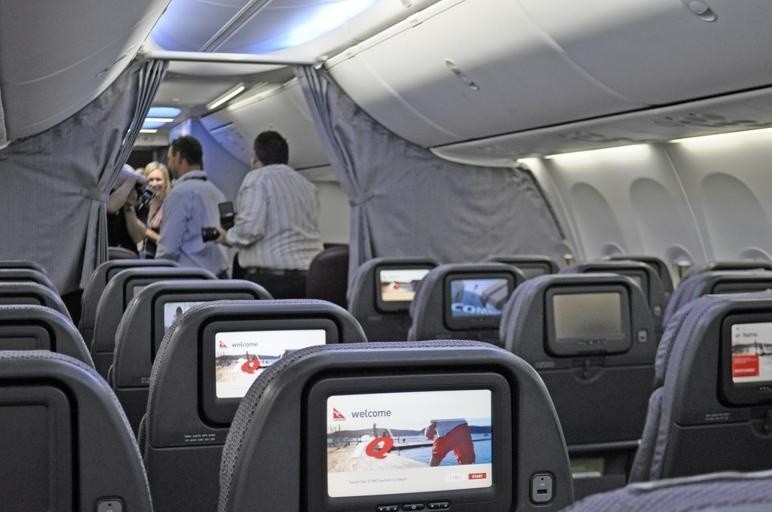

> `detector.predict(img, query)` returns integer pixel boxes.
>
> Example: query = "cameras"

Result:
[200,201,238,242]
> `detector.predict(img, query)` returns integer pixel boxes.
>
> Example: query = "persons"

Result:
[155,135,232,279]
[214,131,326,298]
[107,160,176,261]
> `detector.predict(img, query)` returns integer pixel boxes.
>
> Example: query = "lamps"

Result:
[203,81,248,115]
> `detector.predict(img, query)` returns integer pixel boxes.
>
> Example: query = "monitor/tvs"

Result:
[713,281,772,294]
[546,286,631,353]
[507,262,550,281]
[590,270,649,306]
[721,312,772,403]
[0,278,73,512]
[444,274,515,329]
[151,290,256,359]
[304,372,513,512]
[202,319,340,427]
[375,263,435,312]
[124,278,155,305]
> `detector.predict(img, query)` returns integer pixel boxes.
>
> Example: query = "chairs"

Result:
[1,243,771,512]
